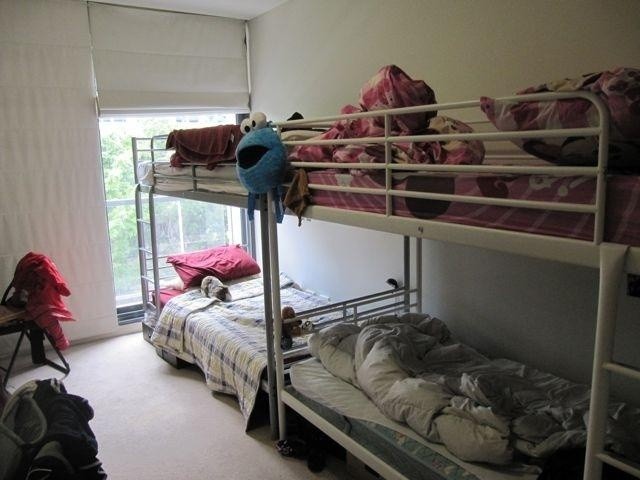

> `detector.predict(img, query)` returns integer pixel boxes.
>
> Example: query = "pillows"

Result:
[474,62,639,172]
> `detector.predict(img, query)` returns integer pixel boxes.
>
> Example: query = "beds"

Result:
[129,91,639,479]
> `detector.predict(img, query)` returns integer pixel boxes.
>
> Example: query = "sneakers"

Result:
[276,438,326,472]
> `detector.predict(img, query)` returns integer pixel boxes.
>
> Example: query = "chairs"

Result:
[0,253,70,391]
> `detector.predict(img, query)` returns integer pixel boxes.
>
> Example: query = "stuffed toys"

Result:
[233,111,291,224]
